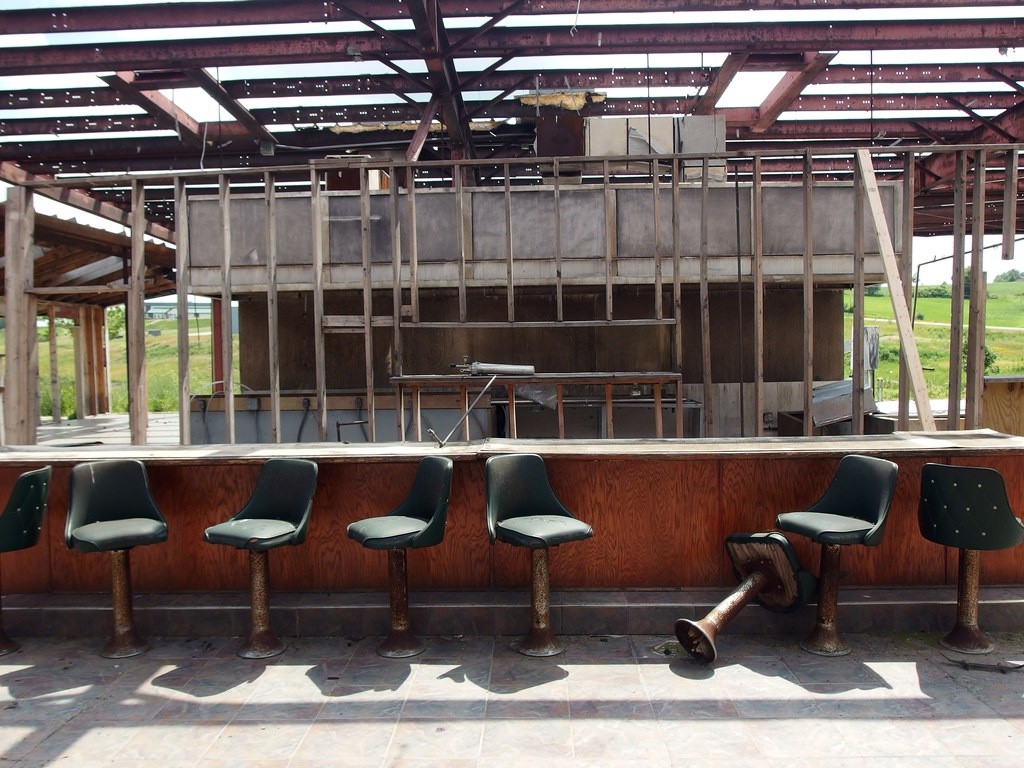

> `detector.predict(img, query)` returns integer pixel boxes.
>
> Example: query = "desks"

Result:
[489,394,706,439]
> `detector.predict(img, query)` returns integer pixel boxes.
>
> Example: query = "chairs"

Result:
[0,465,51,656]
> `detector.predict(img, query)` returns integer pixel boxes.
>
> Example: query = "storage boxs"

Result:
[778,410,852,437]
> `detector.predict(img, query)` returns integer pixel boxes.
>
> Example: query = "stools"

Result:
[674,531,849,665]
[203,459,319,660]
[918,463,1024,654]
[345,457,453,659]
[64,459,169,659]
[485,453,594,657]
[776,454,899,658]
